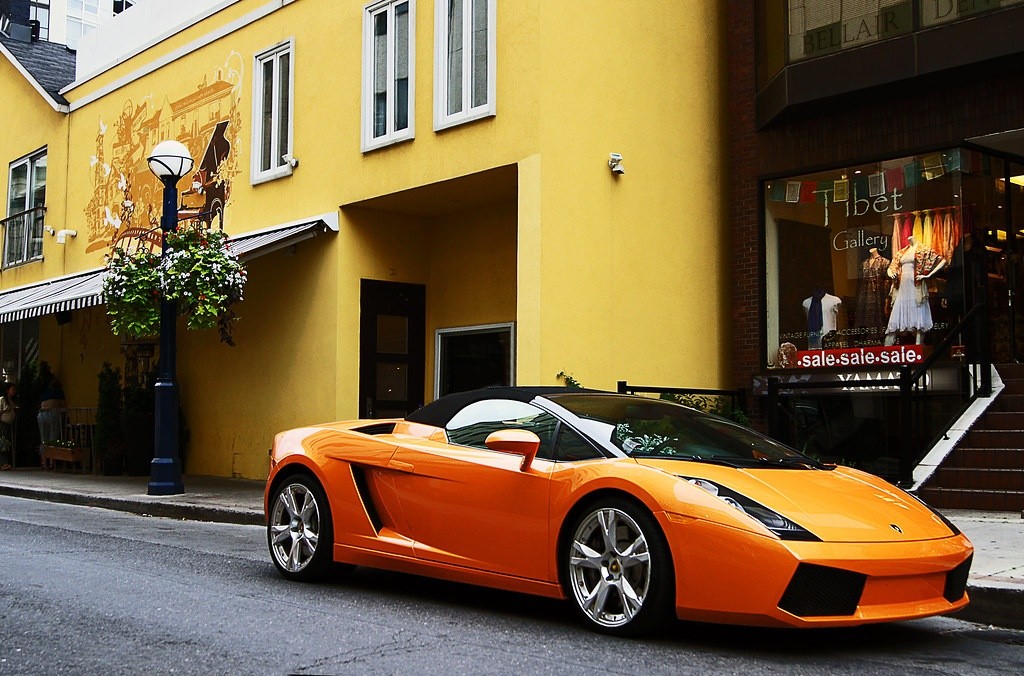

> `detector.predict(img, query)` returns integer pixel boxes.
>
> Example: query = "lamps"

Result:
[608,152,625,175]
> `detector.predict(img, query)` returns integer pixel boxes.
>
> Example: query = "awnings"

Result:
[0,211,340,322]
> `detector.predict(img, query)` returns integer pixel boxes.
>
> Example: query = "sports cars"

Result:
[264,385,975,640]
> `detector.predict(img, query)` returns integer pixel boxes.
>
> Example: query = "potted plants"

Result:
[93,362,126,475]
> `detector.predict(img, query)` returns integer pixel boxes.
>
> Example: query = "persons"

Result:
[852,247,892,347]
[0,382,21,469]
[802,283,841,349]
[886,236,948,347]
[36,380,68,471]
[941,233,994,362]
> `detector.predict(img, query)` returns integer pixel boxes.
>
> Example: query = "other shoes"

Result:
[1,464,11,469]
[42,464,49,470]
[48,464,55,469]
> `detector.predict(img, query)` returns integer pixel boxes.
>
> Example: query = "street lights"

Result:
[147,141,193,490]
